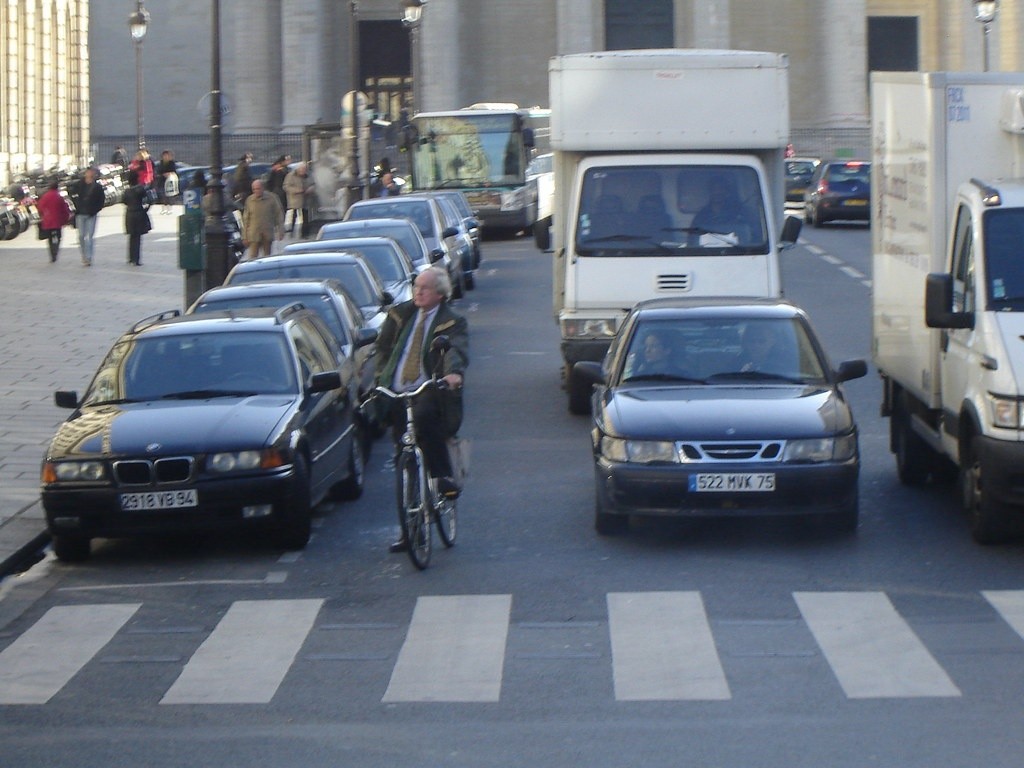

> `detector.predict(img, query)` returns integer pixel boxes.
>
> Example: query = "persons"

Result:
[242,179,284,258]
[631,329,693,376]
[72,167,105,267]
[785,143,795,159]
[370,157,401,199]
[122,172,151,266]
[111,145,176,205]
[186,153,315,239]
[360,267,471,552]
[725,323,796,373]
[687,177,761,247]
[38,181,70,262]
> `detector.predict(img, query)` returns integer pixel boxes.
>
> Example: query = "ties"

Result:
[402,312,428,382]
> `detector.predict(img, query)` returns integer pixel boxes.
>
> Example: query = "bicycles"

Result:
[359,336,457,570]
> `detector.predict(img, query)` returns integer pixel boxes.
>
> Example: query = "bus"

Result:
[412,103,554,235]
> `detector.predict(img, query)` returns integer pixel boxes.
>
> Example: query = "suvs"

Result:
[38,301,364,562]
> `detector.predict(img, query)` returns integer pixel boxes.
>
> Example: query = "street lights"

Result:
[973,0,999,72]
[129,1,147,152]
[402,0,428,116]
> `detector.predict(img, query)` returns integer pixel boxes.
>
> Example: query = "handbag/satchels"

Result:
[165,172,179,197]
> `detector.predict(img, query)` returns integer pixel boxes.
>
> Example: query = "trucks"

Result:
[868,71,1024,545]
[533,49,803,415]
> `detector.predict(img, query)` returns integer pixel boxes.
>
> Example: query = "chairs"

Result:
[222,343,289,387]
[632,196,675,243]
[689,204,762,243]
[590,193,633,237]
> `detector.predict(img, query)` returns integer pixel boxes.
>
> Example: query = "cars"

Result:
[222,250,394,343]
[571,296,869,535]
[182,277,389,438]
[343,191,481,297]
[784,158,820,203]
[154,161,304,203]
[282,237,418,307]
[803,162,873,228]
[317,216,445,274]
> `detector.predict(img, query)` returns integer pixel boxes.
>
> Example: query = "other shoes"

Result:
[161,205,172,215]
[127,261,141,266]
[390,530,425,551]
[438,473,459,498]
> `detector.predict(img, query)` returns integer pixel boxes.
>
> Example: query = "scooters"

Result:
[0,160,129,239]
